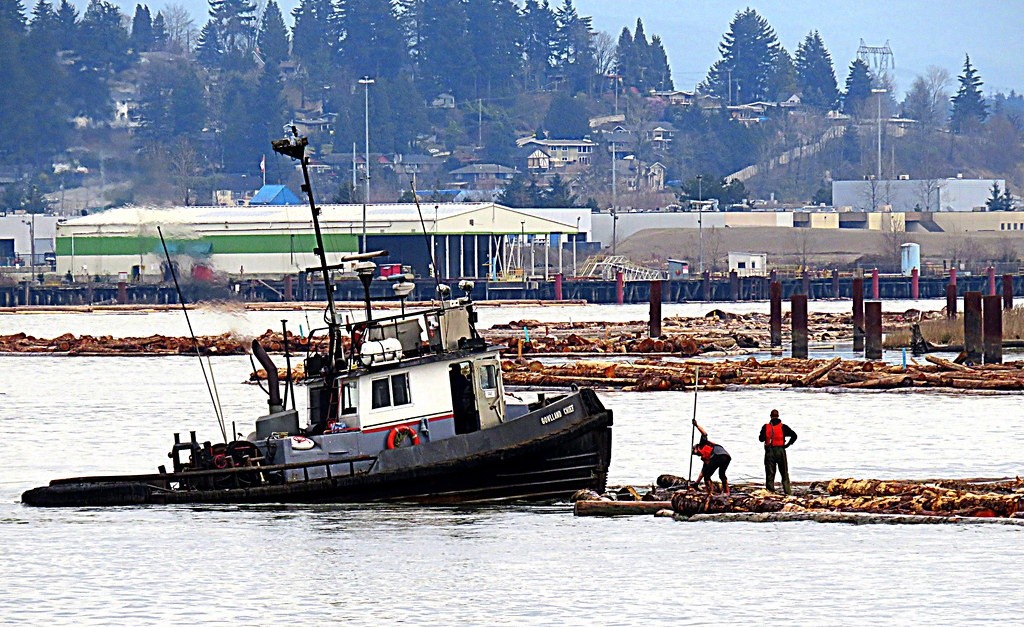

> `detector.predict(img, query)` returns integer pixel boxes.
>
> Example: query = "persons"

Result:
[692,419,732,493]
[758,409,797,495]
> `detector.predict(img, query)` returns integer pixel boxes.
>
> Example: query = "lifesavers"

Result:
[386,425,420,449]
[353,323,368,347]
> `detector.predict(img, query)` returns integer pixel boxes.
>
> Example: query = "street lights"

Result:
[358,75,375,205]
[870,88,887,181]
[694,174,702,275]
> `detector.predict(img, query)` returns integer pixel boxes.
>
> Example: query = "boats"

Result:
[19,122,615,508]
